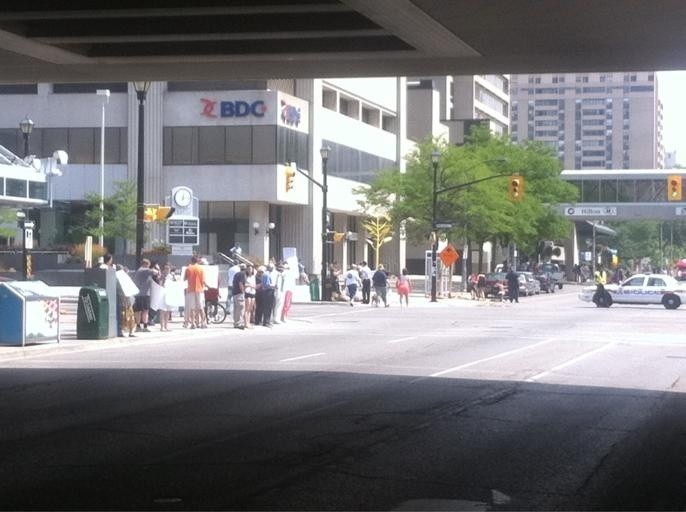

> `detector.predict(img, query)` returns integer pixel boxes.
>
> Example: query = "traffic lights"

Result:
[668,175,682,200]
[285,166,296,192]
[156,205,175,221]
[509,176,523,201]
[335,233,344,242]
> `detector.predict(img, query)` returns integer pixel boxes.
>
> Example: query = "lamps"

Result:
[265,222,275,237]
[252,222,259,236]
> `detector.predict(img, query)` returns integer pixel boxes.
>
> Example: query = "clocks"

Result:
[174,188,191,206]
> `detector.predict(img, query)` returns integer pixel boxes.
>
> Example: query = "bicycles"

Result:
[202,287,227,324]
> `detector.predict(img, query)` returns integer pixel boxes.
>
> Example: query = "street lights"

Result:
[429,151,440,302]
[319,145,332,301]
[130,81,155,270]
[96,89,112,265]
[19,113,35,156]
[592,220,606,283]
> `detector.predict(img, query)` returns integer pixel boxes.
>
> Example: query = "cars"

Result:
[577,272,686,310]
[483,263,568,299]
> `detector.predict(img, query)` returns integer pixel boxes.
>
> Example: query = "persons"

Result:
[182,255,221,329]
[467,268,520,303]
[297,259,411,308]
[572,263,680,309]
[102,254,184,337]
[225,243,295,330]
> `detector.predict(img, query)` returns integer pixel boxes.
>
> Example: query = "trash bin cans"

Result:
[76,286,109,340]
[310,279,319,301]
[0,280,60,346]
